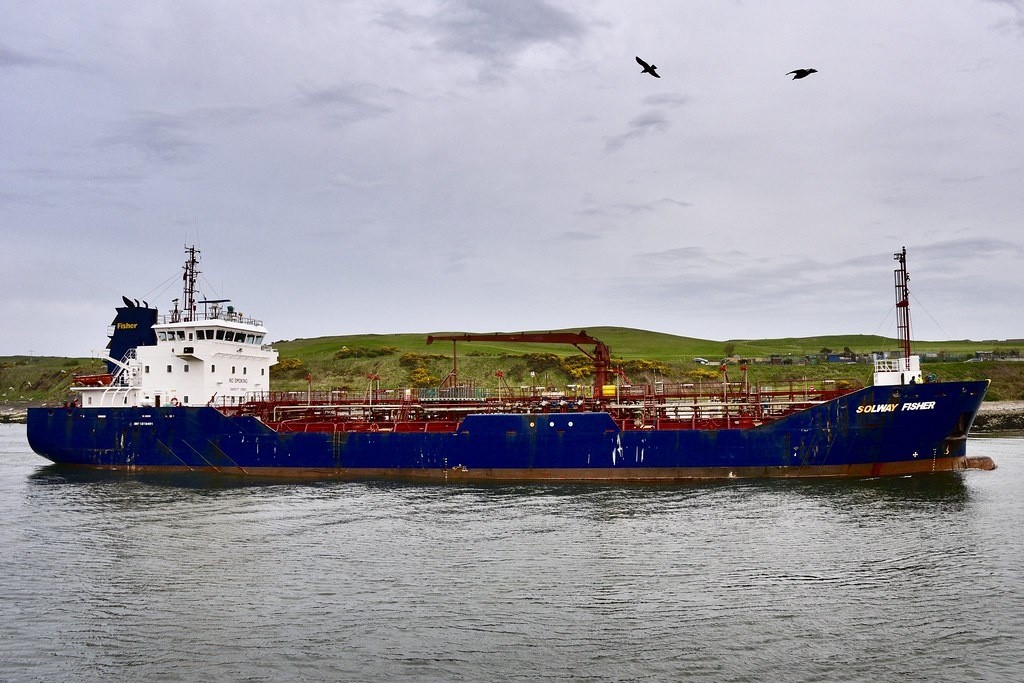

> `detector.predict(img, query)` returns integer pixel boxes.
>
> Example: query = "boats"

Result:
[25,239,999,486]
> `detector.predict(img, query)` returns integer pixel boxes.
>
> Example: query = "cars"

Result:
[693,358,708,363]
[963,357,981,363]
